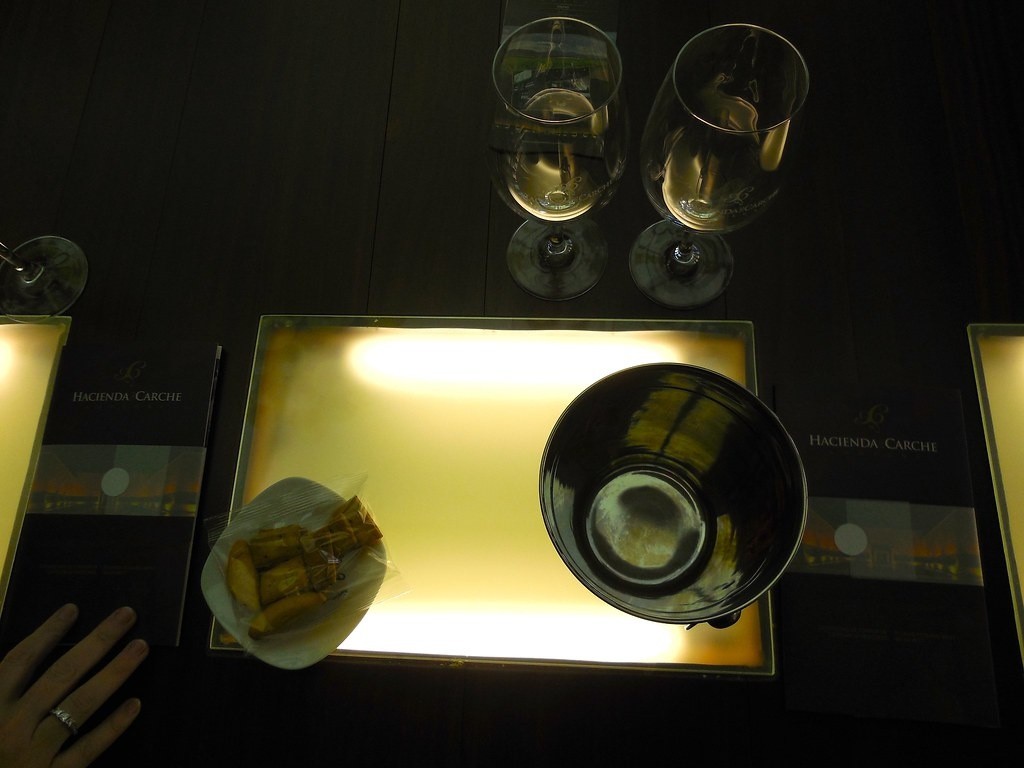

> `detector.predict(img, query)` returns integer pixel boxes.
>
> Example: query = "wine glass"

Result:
[629,24,809,309]
[490,17,632,303]
[0,235,88,323]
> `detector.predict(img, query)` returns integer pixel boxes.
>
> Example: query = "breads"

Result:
[227,495,384,640]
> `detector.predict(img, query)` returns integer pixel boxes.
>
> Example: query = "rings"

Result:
[48,706,80,736]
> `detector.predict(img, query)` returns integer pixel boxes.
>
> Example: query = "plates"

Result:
[199,476,385,672]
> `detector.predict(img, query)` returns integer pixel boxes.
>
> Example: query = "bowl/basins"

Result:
[541,361,810,620]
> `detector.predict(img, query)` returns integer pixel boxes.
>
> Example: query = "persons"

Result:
[0,603,149,768]
